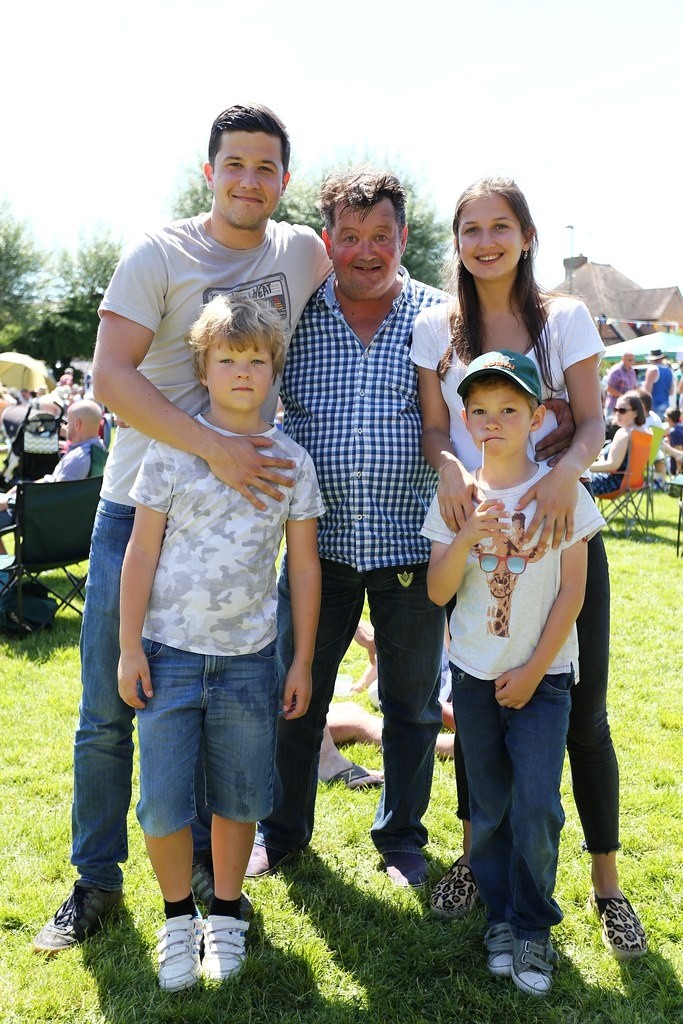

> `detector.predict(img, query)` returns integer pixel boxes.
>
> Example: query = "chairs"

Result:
[0,475,102,634]
[580,426,667,540]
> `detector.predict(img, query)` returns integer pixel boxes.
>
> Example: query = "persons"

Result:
[0,104,683,997]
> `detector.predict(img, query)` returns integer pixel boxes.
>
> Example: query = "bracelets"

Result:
[437,460,464,477]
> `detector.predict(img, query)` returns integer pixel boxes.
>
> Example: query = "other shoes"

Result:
[243,842,300,879]
[430,854,478,918]
[379,852,430,891]
[587,887,648,958]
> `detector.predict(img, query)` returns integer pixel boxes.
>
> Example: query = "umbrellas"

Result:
[0,350,49,392]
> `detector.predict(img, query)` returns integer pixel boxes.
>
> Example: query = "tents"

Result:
[599,331,683,365]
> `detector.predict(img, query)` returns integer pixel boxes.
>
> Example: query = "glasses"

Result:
[613,407,634,414]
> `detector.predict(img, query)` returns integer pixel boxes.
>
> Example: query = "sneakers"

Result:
[190,858,216,906]
[483,921,514,976]
[34,886,124,956]
[201,891,254,985]
[511,936,558,996]
[157,904,204,993]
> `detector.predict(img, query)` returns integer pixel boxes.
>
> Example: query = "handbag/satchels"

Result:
[1,580,59,638]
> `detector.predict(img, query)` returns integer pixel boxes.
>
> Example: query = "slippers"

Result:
[328,761,382,791]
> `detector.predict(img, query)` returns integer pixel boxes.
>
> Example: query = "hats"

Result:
[457,350,542,406]
[645,349,667,361]
